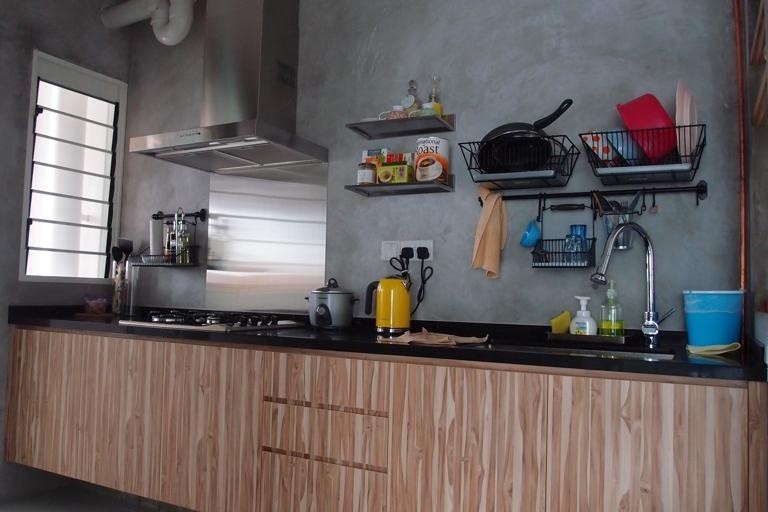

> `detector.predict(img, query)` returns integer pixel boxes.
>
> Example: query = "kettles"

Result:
[365,274,414,335]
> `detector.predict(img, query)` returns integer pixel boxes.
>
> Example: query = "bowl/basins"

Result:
[378,169,393,183]
[581,131,621,168]
[603,128,642,166]
[615,93,676,166]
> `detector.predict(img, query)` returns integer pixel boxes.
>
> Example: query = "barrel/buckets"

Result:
[682,288,744,346]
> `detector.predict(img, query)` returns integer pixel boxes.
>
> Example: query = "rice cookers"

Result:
[305,277,360,330]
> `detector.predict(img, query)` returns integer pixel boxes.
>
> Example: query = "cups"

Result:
[417,156,437,177]
[562,234,586,263]
[379,110,408,121]
[409,108,436,120]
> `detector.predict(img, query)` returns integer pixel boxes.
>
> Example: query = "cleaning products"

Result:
[568,294,598,335]
[599,280,626,336]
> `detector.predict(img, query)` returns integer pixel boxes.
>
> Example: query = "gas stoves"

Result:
[118,309,305,333]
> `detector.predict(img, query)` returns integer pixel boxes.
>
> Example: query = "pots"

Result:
[478,98,574,173]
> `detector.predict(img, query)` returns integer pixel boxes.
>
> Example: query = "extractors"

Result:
[128,1,329,175]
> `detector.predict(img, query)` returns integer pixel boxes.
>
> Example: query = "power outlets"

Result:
[400,239,434,264]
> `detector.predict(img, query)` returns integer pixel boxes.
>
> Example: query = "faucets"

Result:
[590,221,675,338]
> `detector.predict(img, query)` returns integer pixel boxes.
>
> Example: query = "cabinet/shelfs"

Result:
[342,114,455,196]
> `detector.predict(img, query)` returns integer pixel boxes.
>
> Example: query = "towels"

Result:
[469,188,510,280]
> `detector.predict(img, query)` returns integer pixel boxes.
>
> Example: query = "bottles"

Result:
[428,76,440,103]
[170,224,193,264]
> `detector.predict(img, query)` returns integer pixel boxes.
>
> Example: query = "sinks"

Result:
[483,344,675,362]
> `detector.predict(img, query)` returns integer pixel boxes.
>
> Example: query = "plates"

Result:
[415,159,443,183]
[675,78,700,165]
